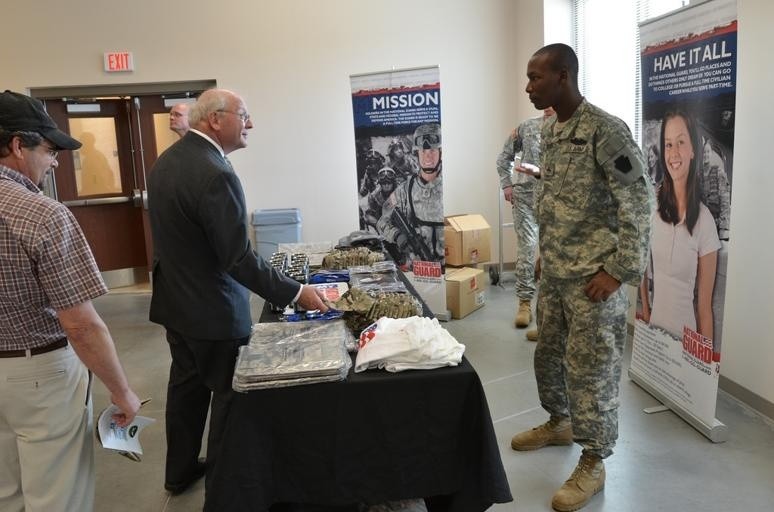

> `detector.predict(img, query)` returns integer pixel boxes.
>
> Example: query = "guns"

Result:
[391,207,435,262]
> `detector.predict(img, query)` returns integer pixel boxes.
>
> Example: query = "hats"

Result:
[0,90,82,150]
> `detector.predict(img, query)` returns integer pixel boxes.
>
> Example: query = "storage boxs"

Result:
[441,211,491,268]
[441,264,488,320]
[441,261,486,271]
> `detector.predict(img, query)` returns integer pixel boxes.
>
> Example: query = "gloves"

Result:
[394,231,414,252]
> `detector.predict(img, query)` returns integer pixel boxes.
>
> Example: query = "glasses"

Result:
[366,150,380,159]
[388,139,403,150]
[415,134,439,146]
[378,170,395,178]
[216,110,250,122]
[39,143,59,160]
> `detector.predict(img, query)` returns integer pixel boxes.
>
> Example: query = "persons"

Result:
[647,144,663,184]
[0,88,155,511]
[142,88,334,510]
[169,103,194,139]
[382,137,418,184]
[511,150,544,343]
[639,107,719,357]
[509,43,654,512]
[699,137,730,241]
[374,122,444,263]
[358,166,402,235]
[356,148,385,199]
[493,106,556,327]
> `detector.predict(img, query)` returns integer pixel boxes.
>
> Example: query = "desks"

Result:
[198,251,514,512]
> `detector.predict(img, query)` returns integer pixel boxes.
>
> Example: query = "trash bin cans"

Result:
[250,208,303,261]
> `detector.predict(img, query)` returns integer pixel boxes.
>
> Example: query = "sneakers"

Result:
[511,416,573,451]
[515,300,532,328]
[526,330,538,340]
[552,455,605,512]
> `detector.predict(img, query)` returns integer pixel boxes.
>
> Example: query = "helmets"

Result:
[366,149,384,161]
[385,139,404,159]
[376,166,396,184]
[412,124,441,156]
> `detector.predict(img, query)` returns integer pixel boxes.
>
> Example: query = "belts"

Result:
[0,337,67,358]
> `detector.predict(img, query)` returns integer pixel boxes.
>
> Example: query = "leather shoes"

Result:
[165,457,206,491]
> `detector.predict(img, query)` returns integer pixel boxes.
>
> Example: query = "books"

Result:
[96,403,156,455]
